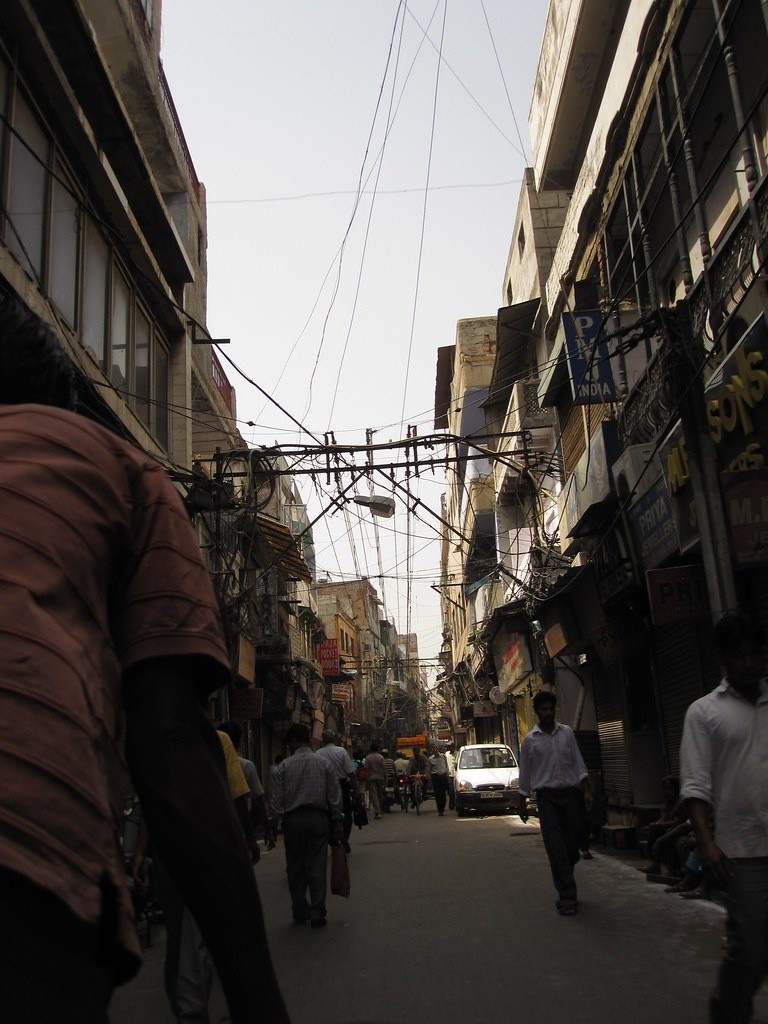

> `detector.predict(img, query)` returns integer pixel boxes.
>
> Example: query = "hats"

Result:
[381,749,388,753]
[446,740,456,747]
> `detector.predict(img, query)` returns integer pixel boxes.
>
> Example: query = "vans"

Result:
[447,740,522,818]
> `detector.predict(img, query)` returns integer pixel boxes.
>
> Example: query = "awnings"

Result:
[434,345,455,430]
[252,514,314,585]
[477,297,541,409]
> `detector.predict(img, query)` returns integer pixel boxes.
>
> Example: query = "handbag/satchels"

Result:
[353,795,368,830]
[331,846,350,898]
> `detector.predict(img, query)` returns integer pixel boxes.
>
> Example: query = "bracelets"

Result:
[656,822,658,826]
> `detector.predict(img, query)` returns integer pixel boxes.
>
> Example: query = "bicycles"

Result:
[381,773,428,815]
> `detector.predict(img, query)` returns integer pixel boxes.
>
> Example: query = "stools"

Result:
[603,824,630,849]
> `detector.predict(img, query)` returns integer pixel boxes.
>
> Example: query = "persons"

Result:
[394,751,409,776]
[268,754,286,801]
[366,743,385,819]
[408,747,428,809]
[679,614,768,1024]
[381,749,405,810]
[315,729,361,854]
[0,295,292,1024]
[444,741,459,810]
[217,720,274,851]
[216,730,261,865]
[268,724,345,929]
[427,743,448,816]
[635,775,685,874]
[351,748,370,809]
[667,857,712,900]
[517,692,593,915]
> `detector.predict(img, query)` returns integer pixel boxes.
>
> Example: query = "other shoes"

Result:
[449,801,455,809]
[341,837,351,853]
[292,913,311,921]
[410,803,415,809]
[401,804,404,809]
[579,849,592,860]
[637,866,660,875]
[424,795,429,801]
[557,900,576,915]
[664,881,697,893]
[311,919,326,929]
[375,814,381,819]
[438,811,443,816]
[678,887,712,899]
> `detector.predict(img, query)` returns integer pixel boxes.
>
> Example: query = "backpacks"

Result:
[353,760,368,781]
